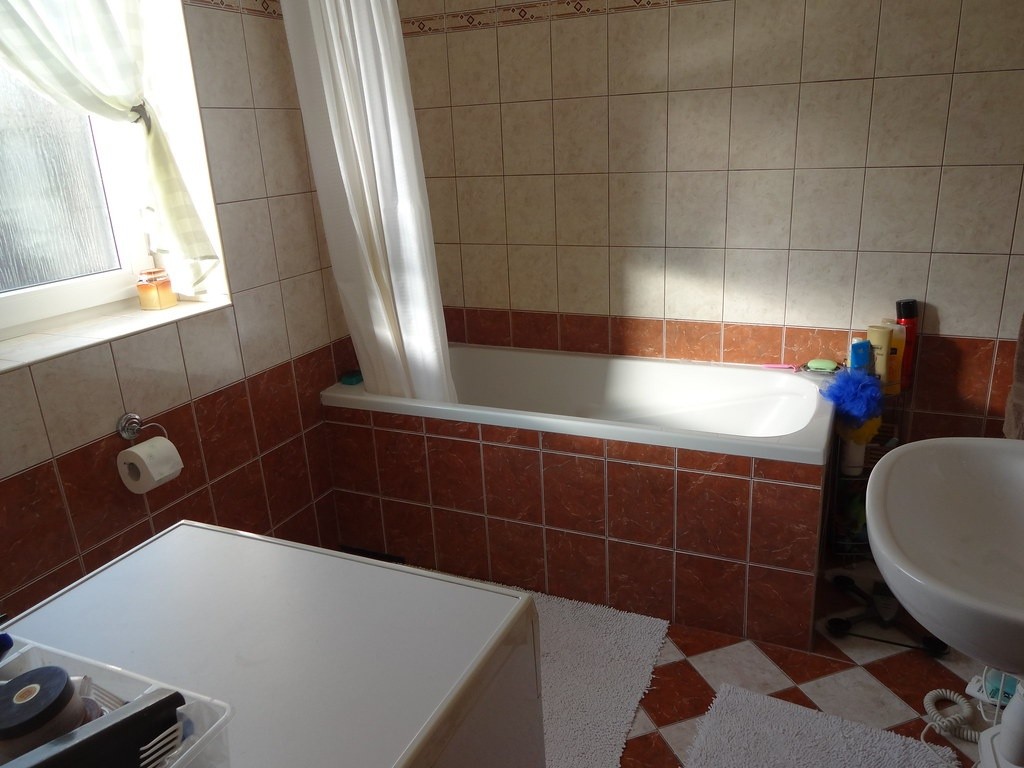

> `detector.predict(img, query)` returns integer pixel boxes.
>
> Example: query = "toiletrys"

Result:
[846,336,866,377]
[966,674,1014,706]
[879,318,907,395]
[875,578,903,630]
[895,298,919,392]
[849,340,872,386]
[863,325,894,398]
[848,554,879,608]
[984,666,1024,695]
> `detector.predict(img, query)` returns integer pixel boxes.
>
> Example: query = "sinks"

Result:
[863,435,1024,675]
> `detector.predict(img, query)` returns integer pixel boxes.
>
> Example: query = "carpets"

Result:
[685,682,963,768]
[388,562,671,767]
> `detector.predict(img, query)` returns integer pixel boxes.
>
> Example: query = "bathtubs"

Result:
[320,334,841,654]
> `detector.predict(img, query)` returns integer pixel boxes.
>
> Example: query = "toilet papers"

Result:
[117,435,183,497]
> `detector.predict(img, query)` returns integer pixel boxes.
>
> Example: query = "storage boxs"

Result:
[0,631,234,768]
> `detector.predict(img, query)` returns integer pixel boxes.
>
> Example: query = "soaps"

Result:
[806,357,838,371]
